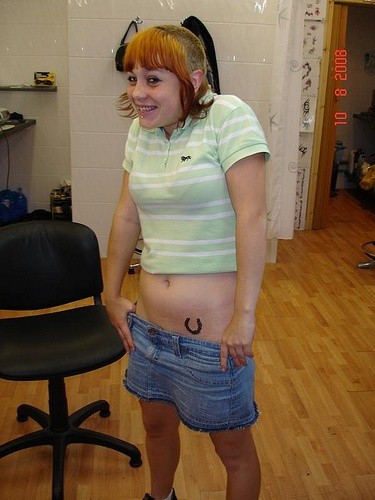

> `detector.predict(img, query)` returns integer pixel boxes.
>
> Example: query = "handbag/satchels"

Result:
[115,20,138,72]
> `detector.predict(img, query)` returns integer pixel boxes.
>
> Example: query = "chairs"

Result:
[0,220,143,500]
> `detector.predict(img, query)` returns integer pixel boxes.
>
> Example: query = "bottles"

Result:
[0,187,27,221]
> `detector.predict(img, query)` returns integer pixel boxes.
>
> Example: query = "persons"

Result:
[104,25,271,500]
